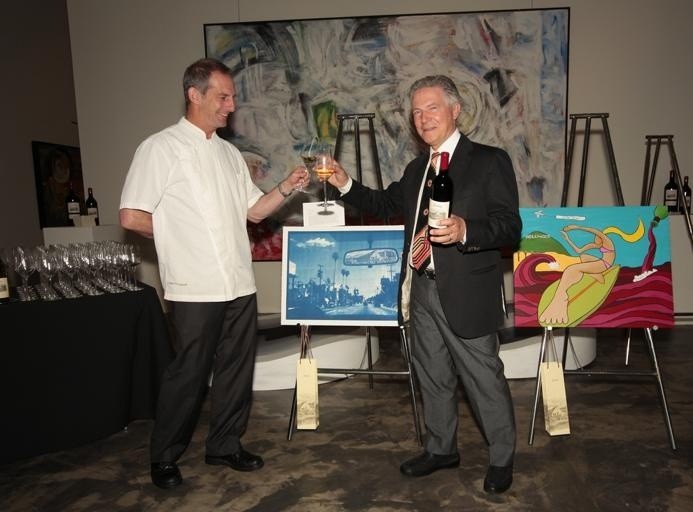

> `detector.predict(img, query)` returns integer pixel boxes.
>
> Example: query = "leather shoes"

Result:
[483,460,514,495]
[399,450,460,479]
[149,457,183,489]
[203,438,265,472]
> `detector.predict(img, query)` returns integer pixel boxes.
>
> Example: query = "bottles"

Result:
[65,180,82,226]
[0,257,9,304]
[427,151,454,245]
[664,170,679,213]
[81,187,100,226]
[678,175,692,215]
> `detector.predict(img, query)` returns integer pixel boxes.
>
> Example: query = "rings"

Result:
[448,236,452,241]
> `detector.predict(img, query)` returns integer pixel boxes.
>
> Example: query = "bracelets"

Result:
[277,182,294,198]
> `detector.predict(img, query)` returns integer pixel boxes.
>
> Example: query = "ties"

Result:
[412,151,441,278]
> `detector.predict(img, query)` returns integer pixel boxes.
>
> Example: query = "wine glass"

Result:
[3,242,140,302]
[314,145,334,208]
[291,135,318,195]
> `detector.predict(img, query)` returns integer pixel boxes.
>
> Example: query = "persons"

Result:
[120,57,311,488]
[313,75,523,495]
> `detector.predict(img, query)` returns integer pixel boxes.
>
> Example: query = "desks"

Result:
[0,266,175,468]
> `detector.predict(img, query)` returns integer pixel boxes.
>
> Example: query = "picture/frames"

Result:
[30,140,85,230]
[278,225,406,327]
[201,8,572,264]
